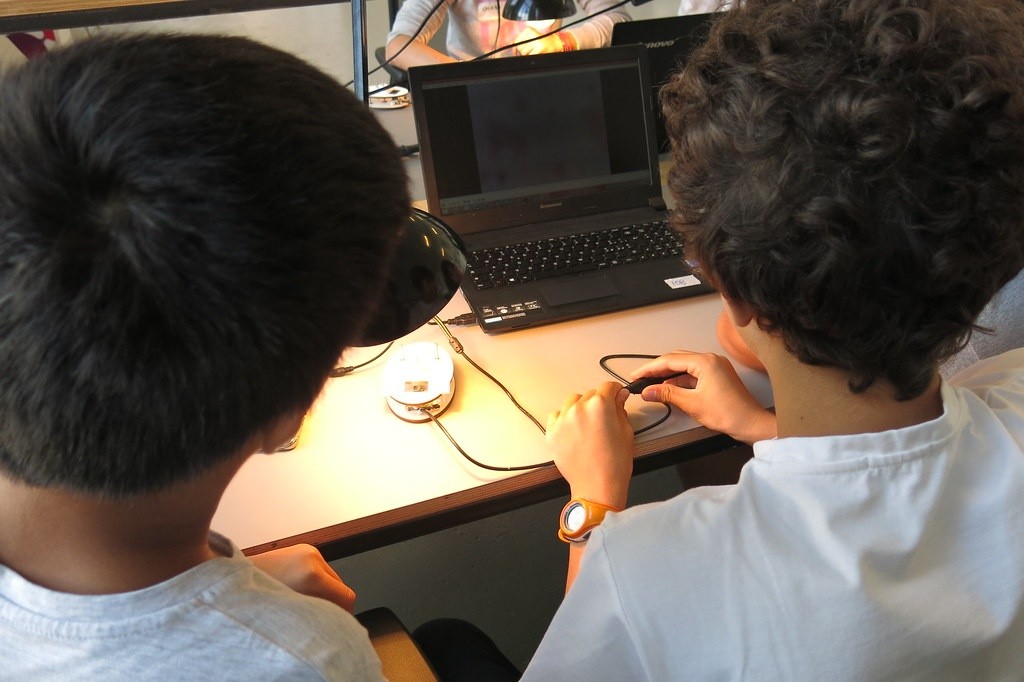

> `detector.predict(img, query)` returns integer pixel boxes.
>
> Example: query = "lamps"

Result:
[504,0,578,32]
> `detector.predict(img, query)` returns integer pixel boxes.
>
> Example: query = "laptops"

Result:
[407,42,719,333]
[612,12,730,153]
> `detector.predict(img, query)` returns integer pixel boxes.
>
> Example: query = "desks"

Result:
[0,1,406,108]
[208,200,775,562]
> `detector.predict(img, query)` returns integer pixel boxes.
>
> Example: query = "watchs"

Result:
[558,497,622,544]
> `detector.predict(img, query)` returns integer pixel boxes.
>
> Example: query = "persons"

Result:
[515,0,1023,682]
[385,0,637,79]
[0,32,416,681]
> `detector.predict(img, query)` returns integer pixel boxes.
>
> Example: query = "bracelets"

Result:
[556,31,575,52]
